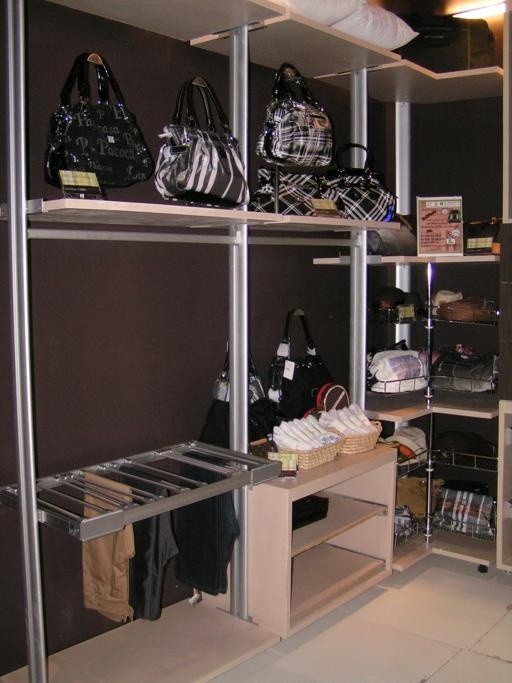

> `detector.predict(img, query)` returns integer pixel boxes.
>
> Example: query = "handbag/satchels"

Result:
[392,13,494,72]
[43,54,154,188]
[153,74,252,209]
[367,287,497,394]
[382,425,497,536]
[464,216,502,253]
[248,143,396,222]
[199,342,278,446]
[255,62,332,167]
[366,212,417,255]
[268,308,331,420]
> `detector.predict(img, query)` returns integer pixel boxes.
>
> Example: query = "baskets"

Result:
[321,420,382,454]
[278,433,345,469]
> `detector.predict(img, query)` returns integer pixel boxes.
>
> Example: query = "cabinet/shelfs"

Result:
[6,0,510,682]
